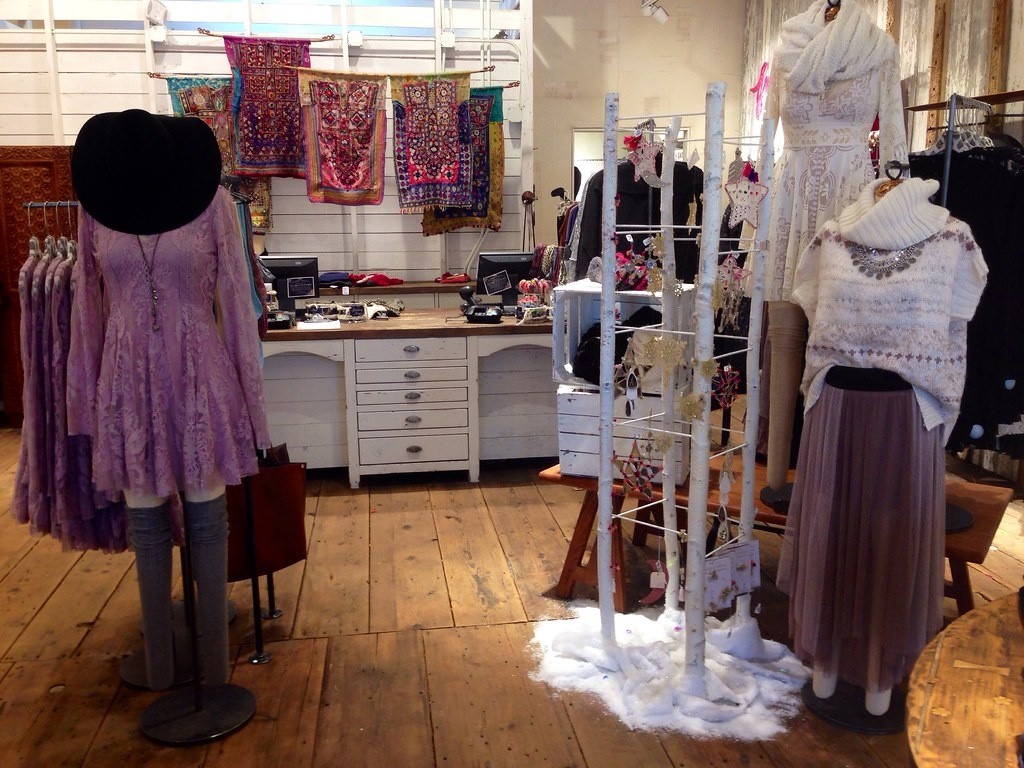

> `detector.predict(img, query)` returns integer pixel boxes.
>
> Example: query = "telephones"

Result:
[267,312,291,329]
[465,305,502,324]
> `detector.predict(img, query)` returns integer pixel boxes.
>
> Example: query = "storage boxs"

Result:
[550,277,698,396]
[557,384,691,488]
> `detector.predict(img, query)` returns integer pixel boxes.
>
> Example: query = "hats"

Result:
[70,109,221,236]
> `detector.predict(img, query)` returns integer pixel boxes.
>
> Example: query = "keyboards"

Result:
[481,303,503,309]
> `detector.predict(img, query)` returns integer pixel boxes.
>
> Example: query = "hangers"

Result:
[907,93,995,156]
[19,200,77,300]
[875,160,903,198]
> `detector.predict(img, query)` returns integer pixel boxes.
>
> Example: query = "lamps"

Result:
[650,4,671,26]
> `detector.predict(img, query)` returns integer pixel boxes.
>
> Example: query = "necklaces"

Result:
[133,233,163,332]
[849,243,925,280]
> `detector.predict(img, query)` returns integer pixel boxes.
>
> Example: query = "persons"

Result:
[65,110,271,688]
[740,0,911,488]
[777,175,988,714]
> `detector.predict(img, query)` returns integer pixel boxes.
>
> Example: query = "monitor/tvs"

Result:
[475,252,535,306]
[259,255,320,310]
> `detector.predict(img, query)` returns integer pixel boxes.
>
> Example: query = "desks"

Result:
[262,307,567,492]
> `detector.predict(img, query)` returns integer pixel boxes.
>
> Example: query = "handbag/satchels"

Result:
[226,442,306,582]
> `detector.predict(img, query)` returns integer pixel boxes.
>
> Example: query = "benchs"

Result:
[538,453,1015,627]
